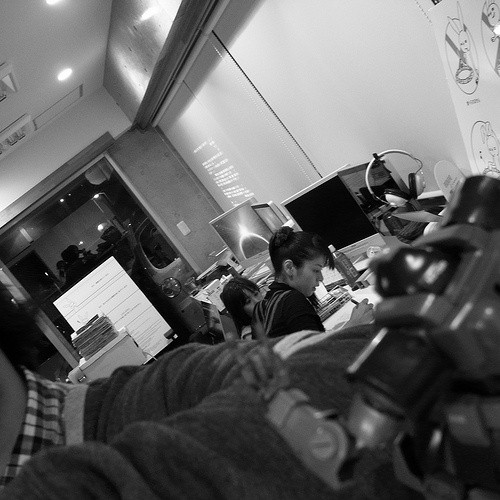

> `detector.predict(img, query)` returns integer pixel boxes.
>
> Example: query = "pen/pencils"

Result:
[351,299,359,306]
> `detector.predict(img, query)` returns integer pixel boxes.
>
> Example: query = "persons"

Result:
[56,244,86,282]
[0,281,395,500]
[251,226,374,340]
[223,278,262,340]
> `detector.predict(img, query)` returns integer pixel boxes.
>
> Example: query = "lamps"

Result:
[19,227,34,245]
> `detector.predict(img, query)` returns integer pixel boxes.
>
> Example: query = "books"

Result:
[206,274,234,315]
[313,284,352,322]
[70,311,119,362]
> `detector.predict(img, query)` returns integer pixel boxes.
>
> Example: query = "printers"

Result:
[68,326,148,384]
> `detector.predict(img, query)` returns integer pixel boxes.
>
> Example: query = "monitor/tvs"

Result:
[280,162,386,272]
[250,201,289,233]
[208,197,275,269]
[336,157,424,237]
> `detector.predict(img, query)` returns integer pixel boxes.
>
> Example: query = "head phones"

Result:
[365,150,425,208]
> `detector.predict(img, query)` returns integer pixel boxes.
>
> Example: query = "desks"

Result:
[67,326,149,389]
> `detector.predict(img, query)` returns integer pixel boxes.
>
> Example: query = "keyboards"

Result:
[320,265,348,291]
